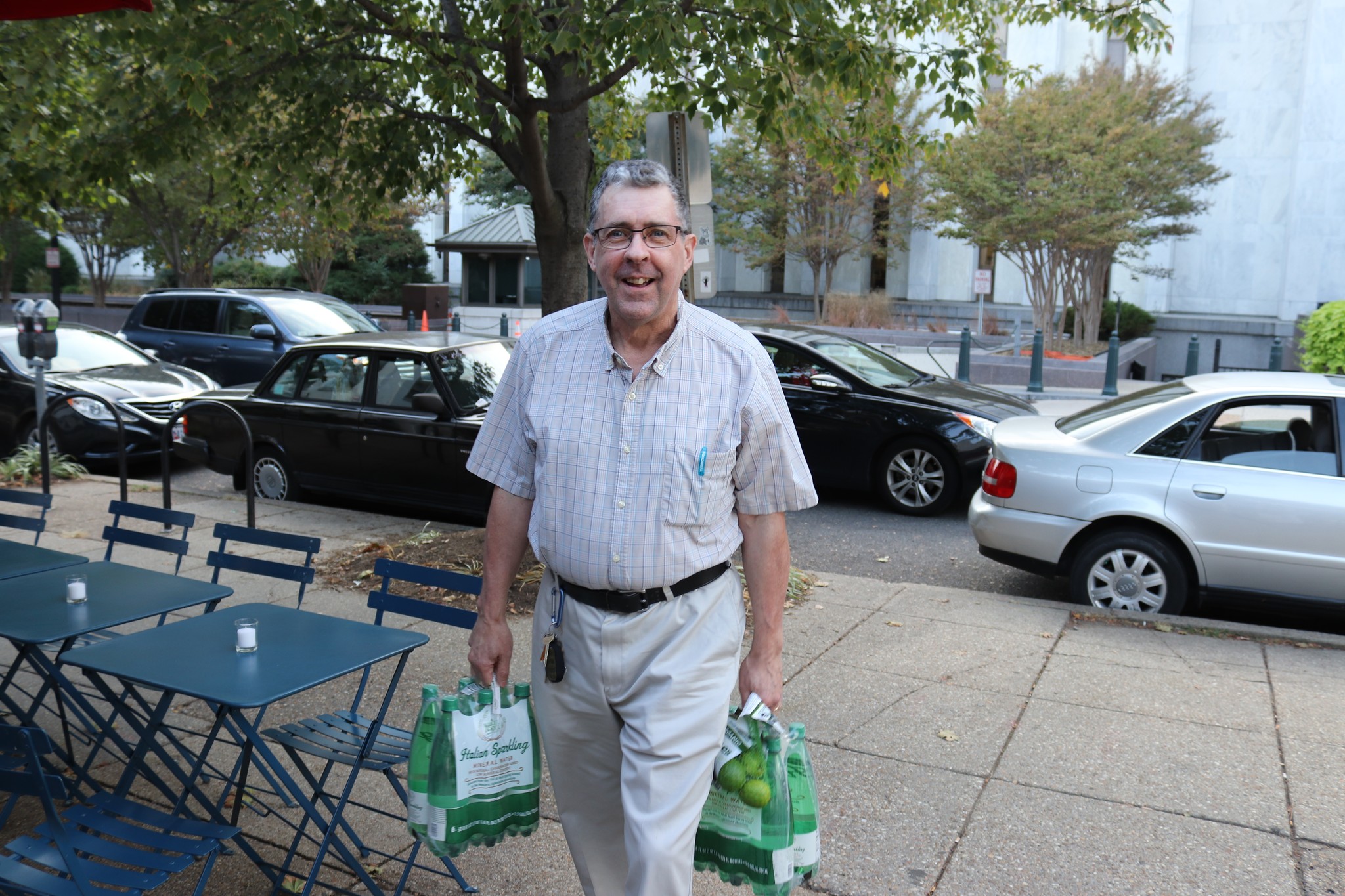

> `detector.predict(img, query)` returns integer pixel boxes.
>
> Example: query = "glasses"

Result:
[592,225,681,250]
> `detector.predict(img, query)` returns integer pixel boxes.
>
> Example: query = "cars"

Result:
[733,321,1042,518]
[967,370,1345,616]
[0,319,223,476]
[176,331,520,526]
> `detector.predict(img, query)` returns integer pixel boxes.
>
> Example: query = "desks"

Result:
[0,538,89,727]
[57,602,429,896]
[0,560,234,808]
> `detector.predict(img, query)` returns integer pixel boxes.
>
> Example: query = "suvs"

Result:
[114,285,465,389]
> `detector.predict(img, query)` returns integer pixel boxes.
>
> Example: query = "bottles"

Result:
[784,722,820,886]
[748,736,794,896]
[428,695,469,858]
[717,866,751,886]
[507,682,541,837]
[694,860,717,872]
[458,677,477,716]
[471,688,505,848]
[407,684,442,841]
[499,686,512,708]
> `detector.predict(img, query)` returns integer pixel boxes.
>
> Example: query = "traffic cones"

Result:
[513,320,522,338]
[420,310,429,332]
[446,312,454,331]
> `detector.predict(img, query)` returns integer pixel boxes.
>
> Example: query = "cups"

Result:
[65,574,88,604]
[234,618,259,653]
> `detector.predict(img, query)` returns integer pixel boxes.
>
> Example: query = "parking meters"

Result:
[15,296,59,509]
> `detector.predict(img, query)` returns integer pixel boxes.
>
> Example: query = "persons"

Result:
[466,157,819,896]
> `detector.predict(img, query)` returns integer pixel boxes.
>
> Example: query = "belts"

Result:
[551,560,731,614]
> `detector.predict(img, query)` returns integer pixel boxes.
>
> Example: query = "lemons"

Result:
[716,753,771,808]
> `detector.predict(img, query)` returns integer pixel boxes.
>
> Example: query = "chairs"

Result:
[1288,417,1318,453]
[0,487,486,896]
